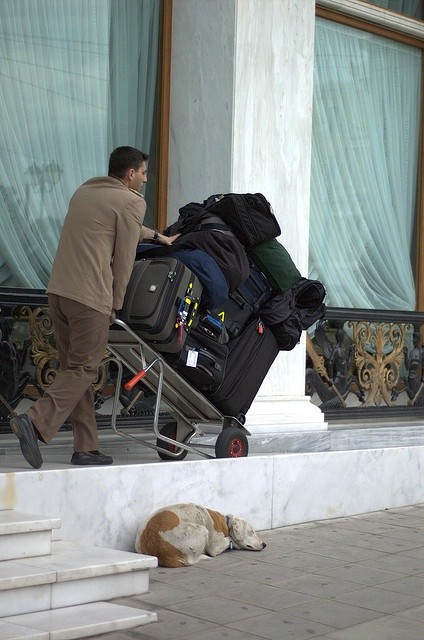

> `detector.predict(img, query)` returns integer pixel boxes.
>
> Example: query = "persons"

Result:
[9,145,181,470]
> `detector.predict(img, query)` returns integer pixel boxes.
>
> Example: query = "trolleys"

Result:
[101,316,252,458]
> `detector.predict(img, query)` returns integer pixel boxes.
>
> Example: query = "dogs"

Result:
[135,503,267,568]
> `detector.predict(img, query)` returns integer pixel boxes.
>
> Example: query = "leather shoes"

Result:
[71,449,113,465]
[10,414,43,469]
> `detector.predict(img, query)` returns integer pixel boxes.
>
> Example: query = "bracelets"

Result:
[153,228,160,242]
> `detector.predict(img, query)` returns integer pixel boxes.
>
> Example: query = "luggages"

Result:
[190,311,235,361]
[237,260,276,313]
[207,289,254,338]
[209,316,280,425]
[117,258,203,354]
[160,332,228,396]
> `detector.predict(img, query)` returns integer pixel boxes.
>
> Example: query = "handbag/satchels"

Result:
[134,242,229,306]
[261,278,328,350]
[251,238,302,292]
[207,187,281,251]
[168,206,252,291]
